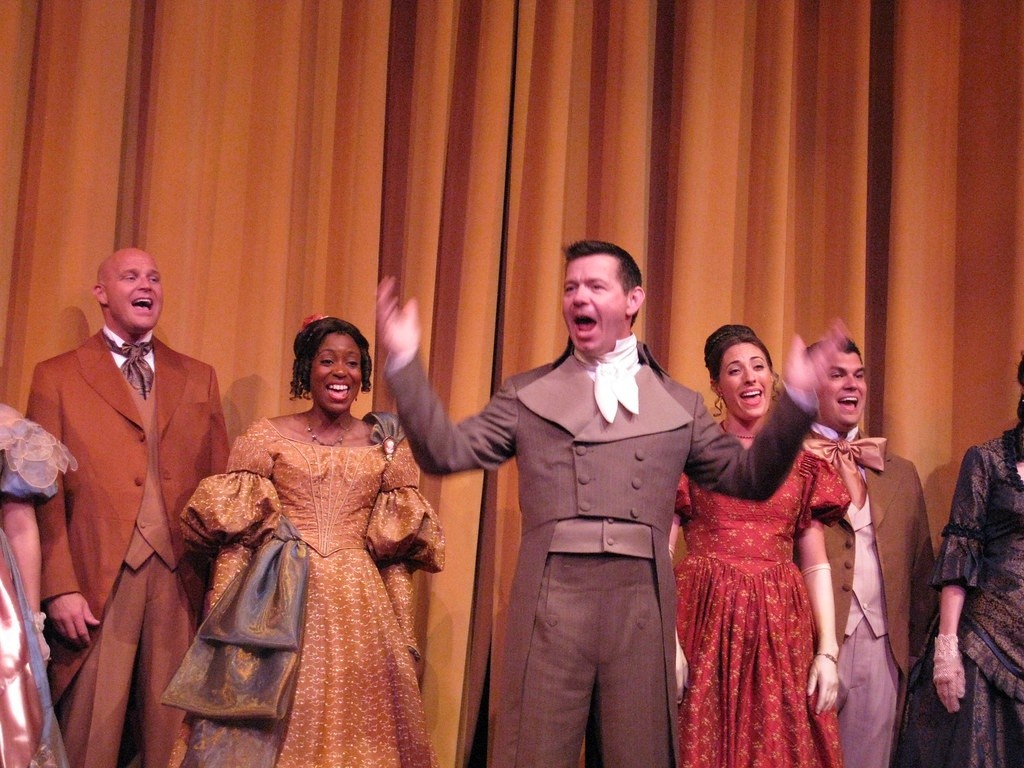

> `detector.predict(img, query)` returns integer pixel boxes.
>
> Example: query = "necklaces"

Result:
[307,411,353,445]
[720,420,755,439]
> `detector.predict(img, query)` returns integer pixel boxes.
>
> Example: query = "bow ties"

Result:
[105,334,153,400]
[802,429,887,512]
[574,348,640,424]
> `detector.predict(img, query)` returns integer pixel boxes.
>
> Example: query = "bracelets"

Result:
[816,653,838,664]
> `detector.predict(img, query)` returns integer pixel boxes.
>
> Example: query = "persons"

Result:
[378,239,849,767]
[0,402,79,768]
[26,245,230,767]
[667,323,852,768]
[803,337,939,768]
[158,313,447,768]
[895,350,1024,768]
